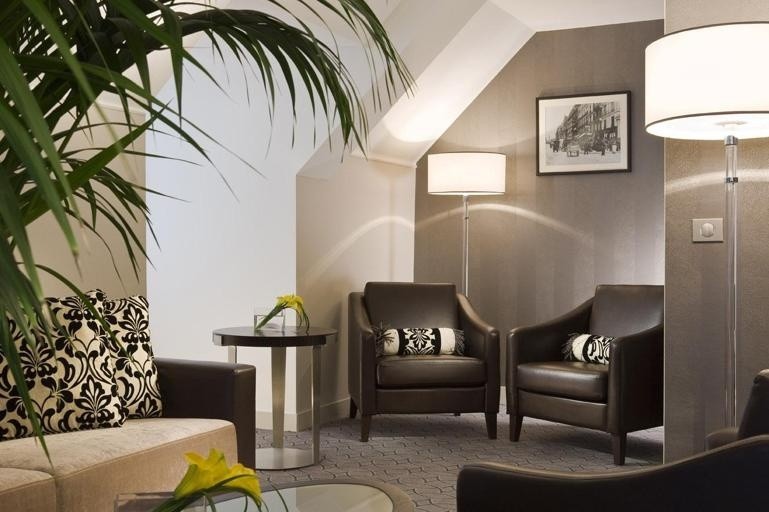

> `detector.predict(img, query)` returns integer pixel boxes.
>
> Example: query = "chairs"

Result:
[505,284,664,467]
[345,281,501,443]
[454,366,769,511]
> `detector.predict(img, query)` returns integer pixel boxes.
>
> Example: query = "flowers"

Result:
[150,449,291,512]
[256,292,310,332]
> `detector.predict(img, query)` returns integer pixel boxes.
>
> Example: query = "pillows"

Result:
[102,294,164,426]
[371,318,468,358]
[562,330,616,367]
[0,287,124,443]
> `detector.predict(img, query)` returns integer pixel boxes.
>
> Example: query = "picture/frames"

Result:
[534,88,634,177]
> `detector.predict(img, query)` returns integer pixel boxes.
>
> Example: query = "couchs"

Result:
[0,355,258,512]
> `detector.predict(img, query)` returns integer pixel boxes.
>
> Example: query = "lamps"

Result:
[424,150,507,300]
[642,20,769,431]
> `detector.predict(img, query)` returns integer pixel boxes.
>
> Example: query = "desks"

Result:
[211,324,338,471]
[184,477,416,512]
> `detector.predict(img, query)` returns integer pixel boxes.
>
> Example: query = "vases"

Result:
[112,486,206,512]
[254,314,286,333]
